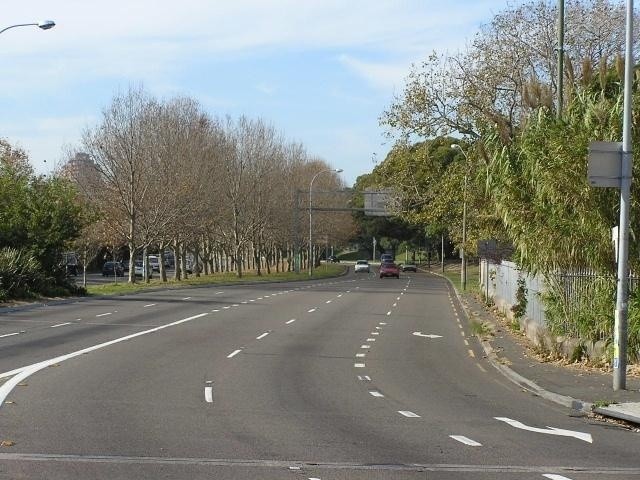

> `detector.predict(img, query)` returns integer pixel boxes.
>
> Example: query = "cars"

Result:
[102,251,203,277]
[379,254,417,279]
[327,256,340,263]
[354,260,370,273]
[59,252,79,276]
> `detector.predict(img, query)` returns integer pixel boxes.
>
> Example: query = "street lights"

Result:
[0,20,55,34]
[309,168,344,275]
[450,143,469,290]
